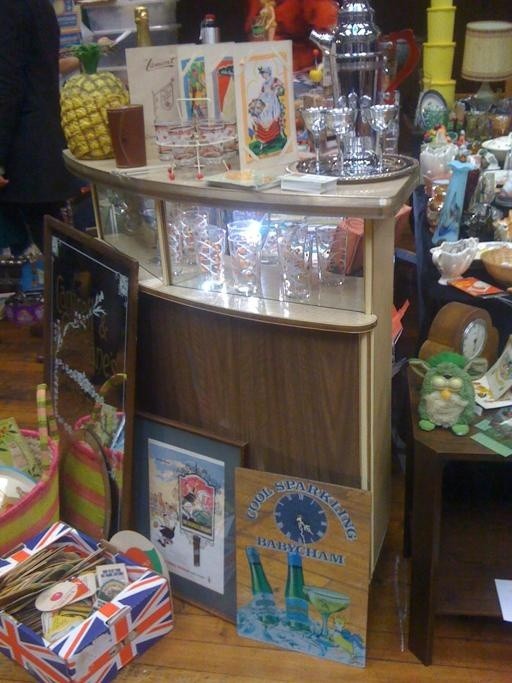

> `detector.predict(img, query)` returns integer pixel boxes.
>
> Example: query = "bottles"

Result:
[246,546,279,624]
[284,552,309,631]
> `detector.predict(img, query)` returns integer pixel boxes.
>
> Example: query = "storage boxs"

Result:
[0,519,174,683]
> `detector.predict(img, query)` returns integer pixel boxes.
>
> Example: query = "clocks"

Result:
[418,300,500,370]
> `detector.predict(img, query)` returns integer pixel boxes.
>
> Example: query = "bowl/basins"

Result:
[480,246,512,284]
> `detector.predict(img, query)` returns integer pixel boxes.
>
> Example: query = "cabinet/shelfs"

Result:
[61,144,419,611]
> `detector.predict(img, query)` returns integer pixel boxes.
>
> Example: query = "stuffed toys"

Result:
[407,351,477,436]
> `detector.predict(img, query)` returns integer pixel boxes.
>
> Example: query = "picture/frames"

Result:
[132,407,248,626]
[41,212,141,540]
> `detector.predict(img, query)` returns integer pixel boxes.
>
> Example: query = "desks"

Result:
[402,366,512,668]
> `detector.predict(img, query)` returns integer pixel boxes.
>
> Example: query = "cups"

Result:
[168,209,347,303]
[105,104,147,170]
[428,236,480,287]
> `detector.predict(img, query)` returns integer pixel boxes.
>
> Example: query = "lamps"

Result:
[461,20,512,111]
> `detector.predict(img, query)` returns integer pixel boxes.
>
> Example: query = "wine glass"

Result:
[301,103,397,175]
[307,586,352,637]
